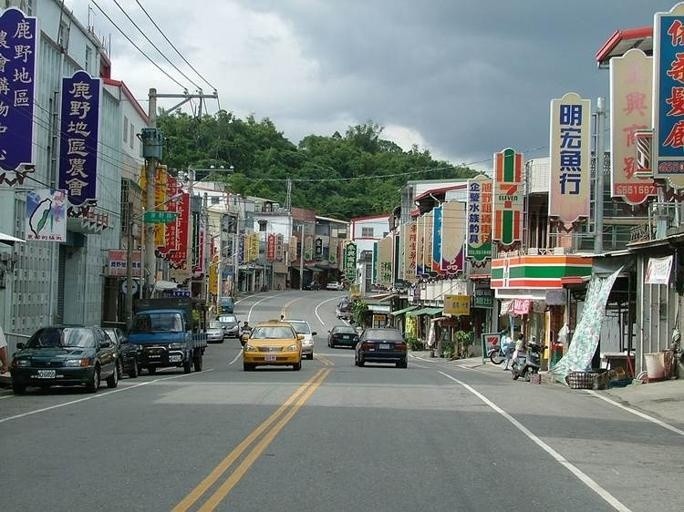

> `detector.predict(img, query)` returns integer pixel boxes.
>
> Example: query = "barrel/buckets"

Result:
[644,351,665,379]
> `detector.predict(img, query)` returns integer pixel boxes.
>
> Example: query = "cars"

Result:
[278,318,317,360]
[8,321,121,396]
[327,324,360,350]
[98,326,133,377]
[242,319,304,371]
[302,278,322,290]
[326,280,344,291]
[352,327,409,369]
[216,313,241,339]
[205,319,226,344]
[220,296,234,314]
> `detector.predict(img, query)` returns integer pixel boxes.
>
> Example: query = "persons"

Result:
[510,334,524,367]
[501,330,515,370]
[242,322,251,330]
[0,328,9,374]
[280,315,284,322]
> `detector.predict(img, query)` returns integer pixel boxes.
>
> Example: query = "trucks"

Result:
[128,306,208,379]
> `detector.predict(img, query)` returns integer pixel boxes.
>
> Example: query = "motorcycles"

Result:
[239,327,252,347]
[489,342,525,364]
[512,342,549,384]
